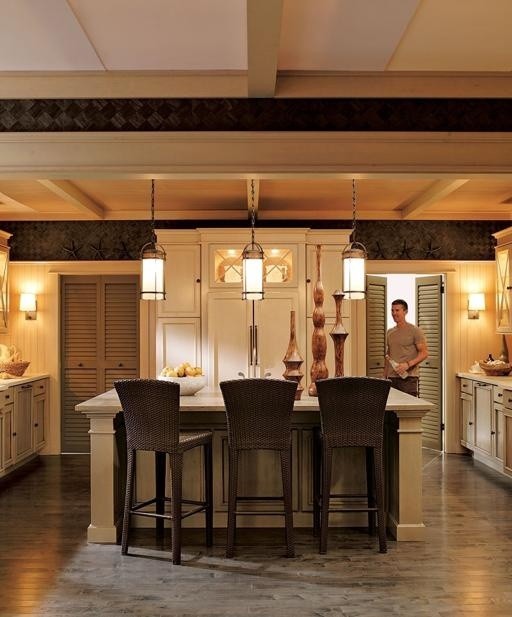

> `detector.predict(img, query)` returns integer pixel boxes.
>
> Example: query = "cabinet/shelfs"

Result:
[0,380,48,484]
[459,380,511,485]
[493,245,512,334]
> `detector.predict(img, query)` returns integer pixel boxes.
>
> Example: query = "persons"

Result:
[383,299,429,399]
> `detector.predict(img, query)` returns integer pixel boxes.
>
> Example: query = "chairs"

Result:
[219,379,298,560]
[315,378,392,555]
[115,380,213,564]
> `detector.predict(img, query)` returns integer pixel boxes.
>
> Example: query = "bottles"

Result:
[487,353,493,361]
[385,354,408,379]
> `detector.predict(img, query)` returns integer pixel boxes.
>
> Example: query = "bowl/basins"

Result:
[156,376,207,395]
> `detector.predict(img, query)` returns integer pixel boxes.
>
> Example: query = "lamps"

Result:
[342,181,367,301]
[241,179,266,303]
[140,180,167,301]
[19,294,37,321]
[467,291,485,319]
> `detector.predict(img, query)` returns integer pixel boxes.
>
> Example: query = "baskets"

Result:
[479,361,511,376]
[1,361,30,378]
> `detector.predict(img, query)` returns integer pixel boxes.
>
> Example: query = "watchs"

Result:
[406,361,411,369]
